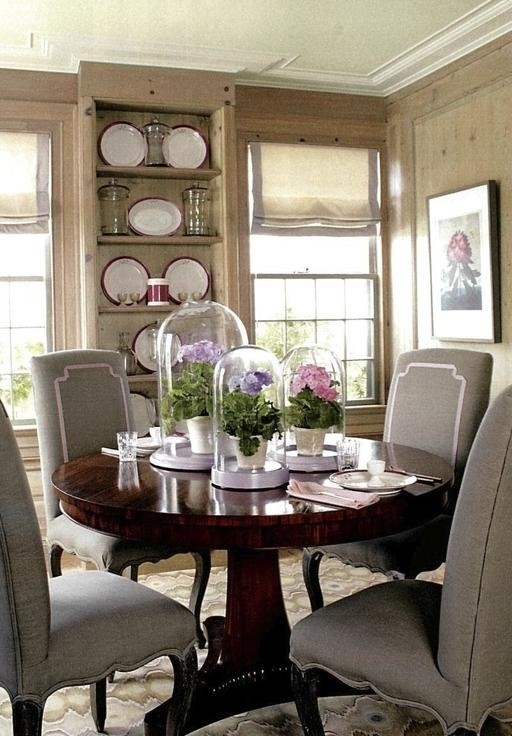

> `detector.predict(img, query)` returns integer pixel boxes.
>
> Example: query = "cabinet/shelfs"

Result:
[76,63,241,427]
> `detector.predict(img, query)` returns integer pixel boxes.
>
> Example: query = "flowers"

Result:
[284,363,345,433]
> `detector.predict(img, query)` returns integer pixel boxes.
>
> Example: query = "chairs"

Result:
[0,399,198,736]
[303,348,493,613]
[30,350,211,649]
[289,384,512,736]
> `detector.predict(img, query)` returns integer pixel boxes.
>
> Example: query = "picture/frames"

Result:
[425,180,501,343]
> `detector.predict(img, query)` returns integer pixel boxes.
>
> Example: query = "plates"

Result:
[95,113,220,374]
[134,426,189,451]
[330,469,417,496]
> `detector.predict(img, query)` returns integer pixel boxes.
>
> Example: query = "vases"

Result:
[290,424,333,456]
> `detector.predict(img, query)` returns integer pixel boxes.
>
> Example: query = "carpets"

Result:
[0,554,511,736]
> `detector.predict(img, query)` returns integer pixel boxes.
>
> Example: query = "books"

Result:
[101,445,128,459]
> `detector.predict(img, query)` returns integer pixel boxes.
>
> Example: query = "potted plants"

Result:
[216,384,284,469]
[160,361,216,455]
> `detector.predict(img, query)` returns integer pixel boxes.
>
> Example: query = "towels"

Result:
[286,479,380,510]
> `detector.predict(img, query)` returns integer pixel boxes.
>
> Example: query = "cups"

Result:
[115,430,140,462]
[366,460,389,488]
[112,463,142,495]
[337,437,363,469]
